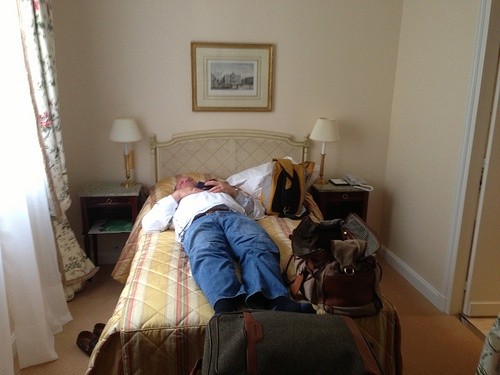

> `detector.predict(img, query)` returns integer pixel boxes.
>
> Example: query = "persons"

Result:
[141,175,317,313]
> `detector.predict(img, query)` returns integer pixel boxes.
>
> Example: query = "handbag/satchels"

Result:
[282,212,383,317]
[190,309,385,375]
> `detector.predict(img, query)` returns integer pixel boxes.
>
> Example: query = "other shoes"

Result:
[93,323,106,336]
[77,331,99,357]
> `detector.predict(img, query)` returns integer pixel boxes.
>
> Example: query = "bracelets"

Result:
[232,186,238,196]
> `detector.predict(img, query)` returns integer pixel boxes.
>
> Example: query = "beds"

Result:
[86,129,402,375]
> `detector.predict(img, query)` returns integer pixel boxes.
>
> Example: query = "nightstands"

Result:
[77,179,142,283]
[310,176,368,223]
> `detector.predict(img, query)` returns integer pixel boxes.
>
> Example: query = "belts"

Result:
[193,209,229,221]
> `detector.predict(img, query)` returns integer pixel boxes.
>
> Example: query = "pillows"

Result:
[150,174,221,205]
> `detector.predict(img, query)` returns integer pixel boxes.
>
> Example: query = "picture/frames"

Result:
[190,41,275,112]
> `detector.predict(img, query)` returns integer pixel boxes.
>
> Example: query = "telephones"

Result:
[343,174,368,186]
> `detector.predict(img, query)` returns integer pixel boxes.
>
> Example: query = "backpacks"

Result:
[256,158,324,223]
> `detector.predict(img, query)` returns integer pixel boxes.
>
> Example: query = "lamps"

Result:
[308,116,341,185]
[110,118,142,188]
